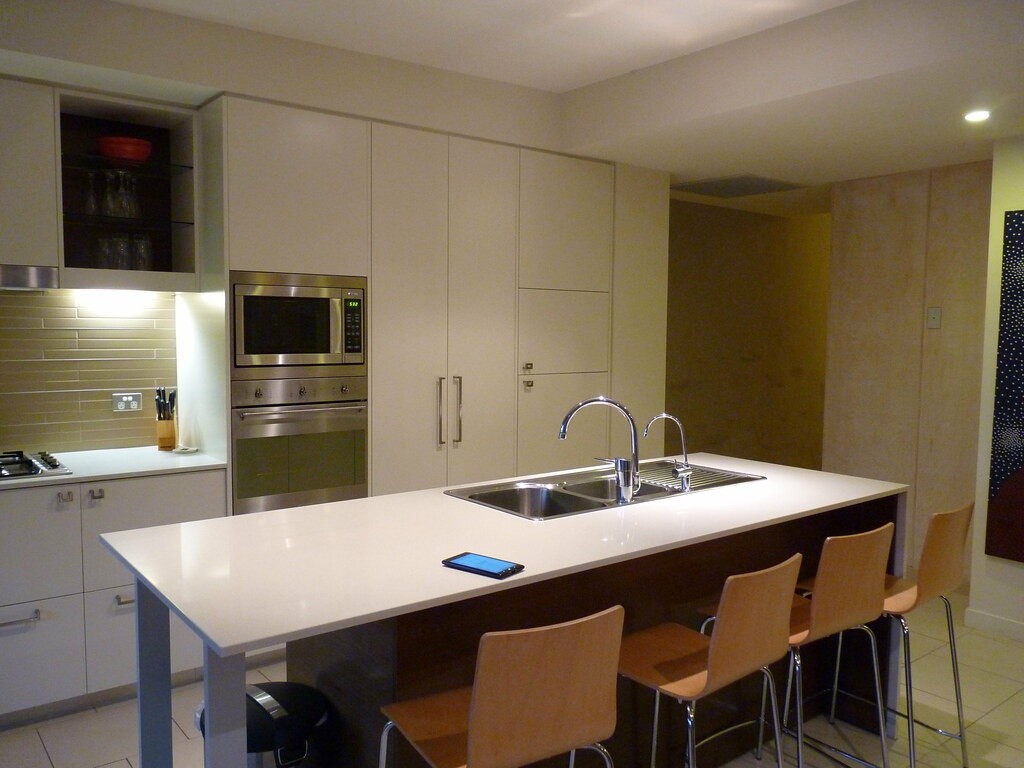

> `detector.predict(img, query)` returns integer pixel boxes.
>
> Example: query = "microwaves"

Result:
[233,283,365,367]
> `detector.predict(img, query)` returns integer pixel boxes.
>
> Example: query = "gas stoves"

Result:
[0,449,73,481]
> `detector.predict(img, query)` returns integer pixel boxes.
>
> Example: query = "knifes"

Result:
[155,387,176,420]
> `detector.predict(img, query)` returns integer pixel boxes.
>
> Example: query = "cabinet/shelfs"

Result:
[0,74,617,730]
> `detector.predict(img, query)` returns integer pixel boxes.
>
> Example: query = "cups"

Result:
[93,231,152,270]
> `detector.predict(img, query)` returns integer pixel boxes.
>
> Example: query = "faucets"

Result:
[643,413,692,493]
[557,396,641,505]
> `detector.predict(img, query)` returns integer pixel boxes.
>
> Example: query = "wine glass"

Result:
[77,167,142,218]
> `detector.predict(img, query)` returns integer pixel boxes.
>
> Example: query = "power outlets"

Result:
[112,392,142,412]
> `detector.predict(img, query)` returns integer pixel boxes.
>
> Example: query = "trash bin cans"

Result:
[195,681,343,768]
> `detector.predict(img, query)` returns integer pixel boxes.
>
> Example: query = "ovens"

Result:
[230,360,373,515]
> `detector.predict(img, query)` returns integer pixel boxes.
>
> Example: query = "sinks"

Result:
[561,477,682,503]
[442,480,612,522]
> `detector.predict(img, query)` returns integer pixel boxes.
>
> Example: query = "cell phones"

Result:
[443,552,525,579]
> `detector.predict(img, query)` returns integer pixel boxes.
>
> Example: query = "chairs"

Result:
[696,521,896,768]
[618,552,803,768]
[796,500,976,768]
[377,605,625,768]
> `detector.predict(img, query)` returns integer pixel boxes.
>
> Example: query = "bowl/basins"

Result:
[98,135,152,160]
